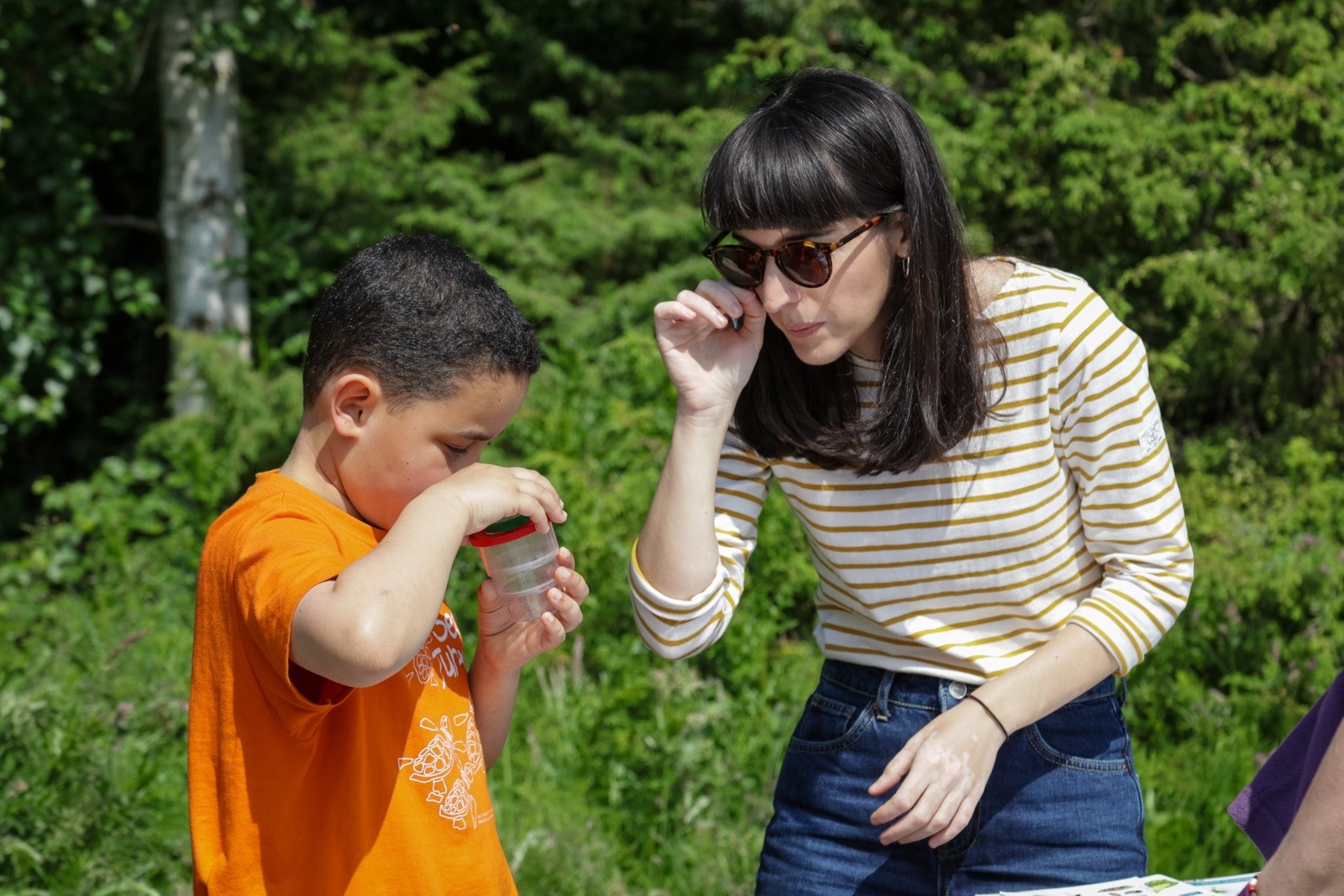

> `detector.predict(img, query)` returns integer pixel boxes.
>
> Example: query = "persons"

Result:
[1228,665,1344,896]
[184,226,588,896]
[628,66,1195,896]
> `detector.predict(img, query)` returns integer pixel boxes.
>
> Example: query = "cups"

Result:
[468,514,563,621]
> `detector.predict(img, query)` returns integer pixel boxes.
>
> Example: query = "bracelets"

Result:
[967,694,1009,744]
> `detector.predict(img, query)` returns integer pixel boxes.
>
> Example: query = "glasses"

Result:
[701,204,906,290]
